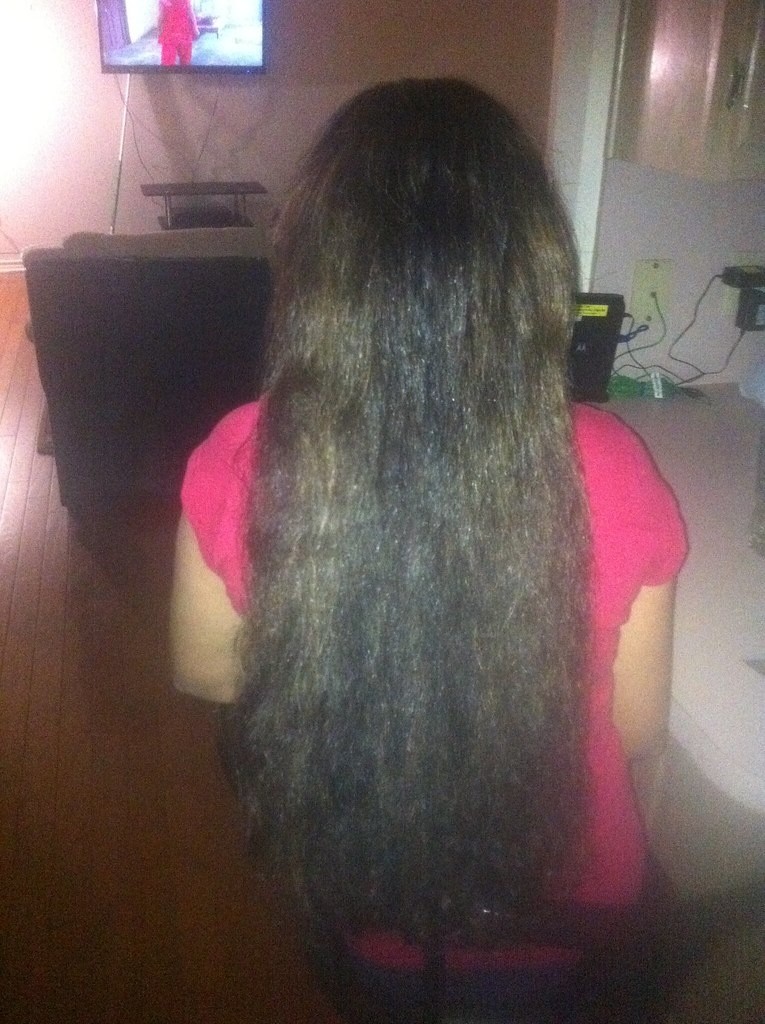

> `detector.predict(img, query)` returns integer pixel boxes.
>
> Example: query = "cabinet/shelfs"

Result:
[141,181,268,230]
[605,0,765,180]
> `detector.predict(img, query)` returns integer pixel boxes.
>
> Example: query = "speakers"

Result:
[565,293,626,402]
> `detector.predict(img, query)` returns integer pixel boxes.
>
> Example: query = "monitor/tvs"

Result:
[96,0,268,75]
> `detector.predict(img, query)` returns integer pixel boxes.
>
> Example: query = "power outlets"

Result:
[720,253,764,324]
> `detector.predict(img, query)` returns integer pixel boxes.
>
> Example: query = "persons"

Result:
[171,80,692,1023]
[158,0,200,66]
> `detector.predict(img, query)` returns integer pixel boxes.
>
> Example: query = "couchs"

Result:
[20,229,271,508]
